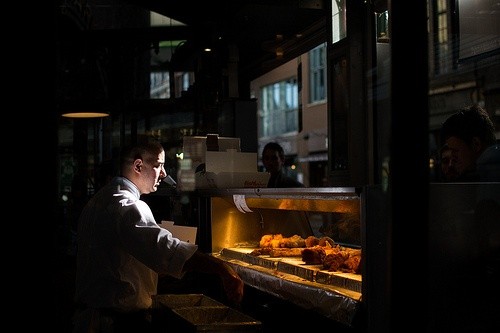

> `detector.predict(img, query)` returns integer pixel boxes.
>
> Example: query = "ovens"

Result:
[198,186,367,333]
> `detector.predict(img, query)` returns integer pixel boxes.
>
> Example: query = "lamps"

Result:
[59,31,113,121]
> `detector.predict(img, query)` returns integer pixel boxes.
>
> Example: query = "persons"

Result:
[440,100,500,253]
[261,141,302,187]
[433,142,465,187]
[71,129,244,331]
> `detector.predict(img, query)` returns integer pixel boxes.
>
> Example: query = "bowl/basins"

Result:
[205,170,272,192]
[150,292,262,332]
